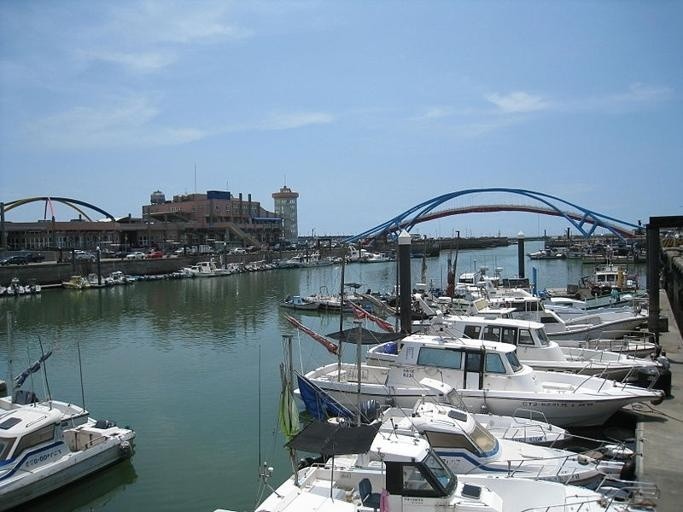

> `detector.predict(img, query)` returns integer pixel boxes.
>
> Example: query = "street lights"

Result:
[357,238,362,259]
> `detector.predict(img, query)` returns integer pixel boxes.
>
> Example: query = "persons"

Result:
[655,349,671,376]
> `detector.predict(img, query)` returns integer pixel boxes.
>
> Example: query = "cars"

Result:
[174,246,189,255]
[101,248,163,260]
[25,252,46,263]
[1,255,28,266]
[68,248,96,261]
[230,248,246,254]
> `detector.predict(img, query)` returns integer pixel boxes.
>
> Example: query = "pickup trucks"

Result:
[246,246,261,253]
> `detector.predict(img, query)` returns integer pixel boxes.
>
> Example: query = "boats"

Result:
[296,393,628,490]
[0,335,137,512]
[367,310,663,385]
[254,431,662,512]
[60,269,138,288]
[292,332,666,436]
[0,277,44,298]
[252,228,667,358]
[176,261,260,278]
[365,377,573,449]
[278,265,652,332]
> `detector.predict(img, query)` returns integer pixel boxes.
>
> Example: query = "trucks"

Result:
[191,245,215,255]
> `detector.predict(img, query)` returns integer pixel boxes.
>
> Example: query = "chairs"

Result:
[358,477,381,512]
